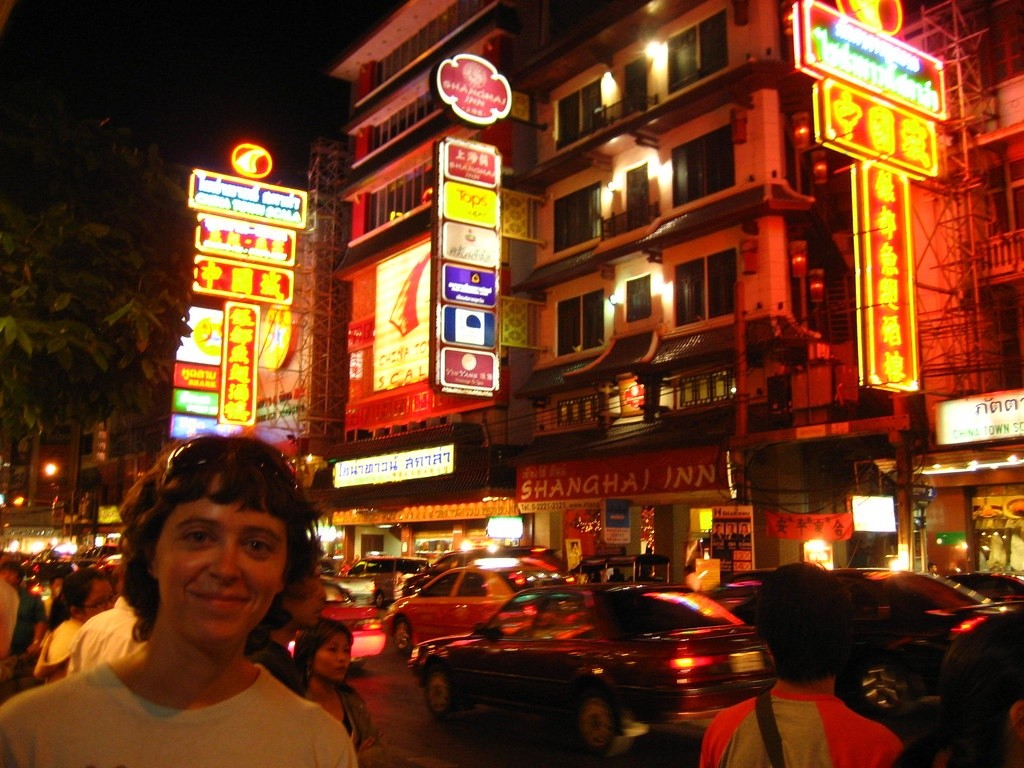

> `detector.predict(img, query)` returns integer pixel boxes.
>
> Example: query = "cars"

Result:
[714,558,1024,722]
[21,540,126,596]
[411,580,780,760]
[283,545,673,675]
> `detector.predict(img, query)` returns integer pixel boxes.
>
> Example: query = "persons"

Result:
[701,558,1024,767]
[0,435,382,768]
[714,524,750,545]
[584,564,659,583]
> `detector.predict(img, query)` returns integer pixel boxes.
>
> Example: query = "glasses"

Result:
[81,591,116,610]
[160,435,298,494]
